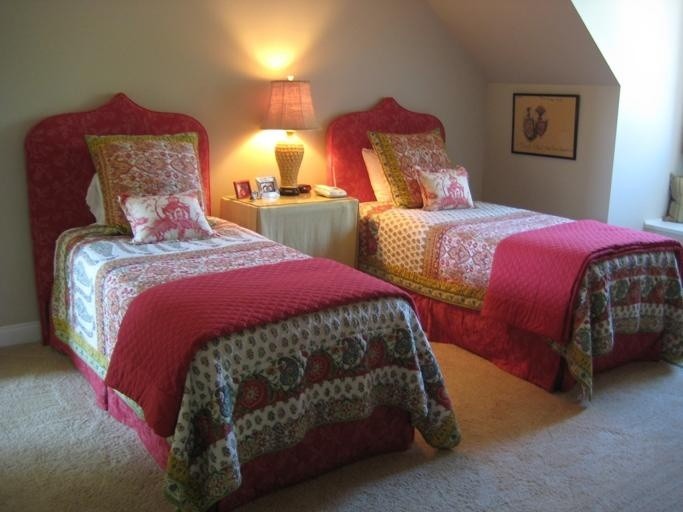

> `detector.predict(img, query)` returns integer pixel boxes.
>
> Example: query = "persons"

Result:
[262,186,274,193]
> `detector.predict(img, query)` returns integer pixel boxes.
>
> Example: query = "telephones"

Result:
[314,185,347,197]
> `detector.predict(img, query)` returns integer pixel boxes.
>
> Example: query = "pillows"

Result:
[361,127,474,211]
[663,174,683,223]
[85,132,220,245]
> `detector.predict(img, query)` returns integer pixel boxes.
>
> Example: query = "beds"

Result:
[325,98,683,391]
[24,93,415,511]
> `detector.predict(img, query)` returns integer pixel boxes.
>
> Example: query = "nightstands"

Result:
[220,191,359,268]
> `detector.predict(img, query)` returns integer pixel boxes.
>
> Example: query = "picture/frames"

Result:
[234,182,252,199]
[257,177,280,198]
[511,93,580,160]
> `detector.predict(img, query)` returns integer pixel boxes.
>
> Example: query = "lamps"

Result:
[260,76,320,196]
